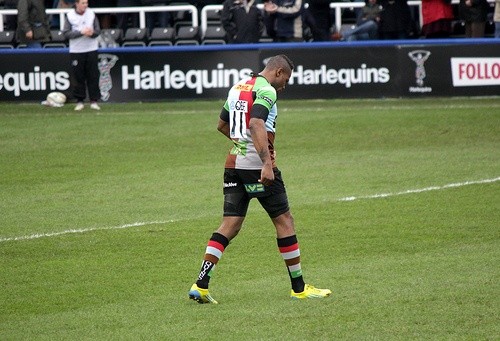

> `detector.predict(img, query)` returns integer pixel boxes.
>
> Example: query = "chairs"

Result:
[0,0,500,49]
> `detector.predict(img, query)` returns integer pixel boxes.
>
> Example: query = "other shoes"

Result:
[74,103,84,110]
[91,103,100,110]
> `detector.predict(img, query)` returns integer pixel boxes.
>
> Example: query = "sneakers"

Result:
[290,285,331,299]
[188,284,218,305]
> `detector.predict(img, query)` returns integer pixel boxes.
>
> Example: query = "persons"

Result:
[460,0,491,40]
[221,0,265,44]
[265,1,301,42]
[379,0,418,38]
[303,1,337,41]
[188,54,331,305]
[332,0,382,43]
[16,1,52,48]
[63,0,103,110]
[420,0,454,39]
